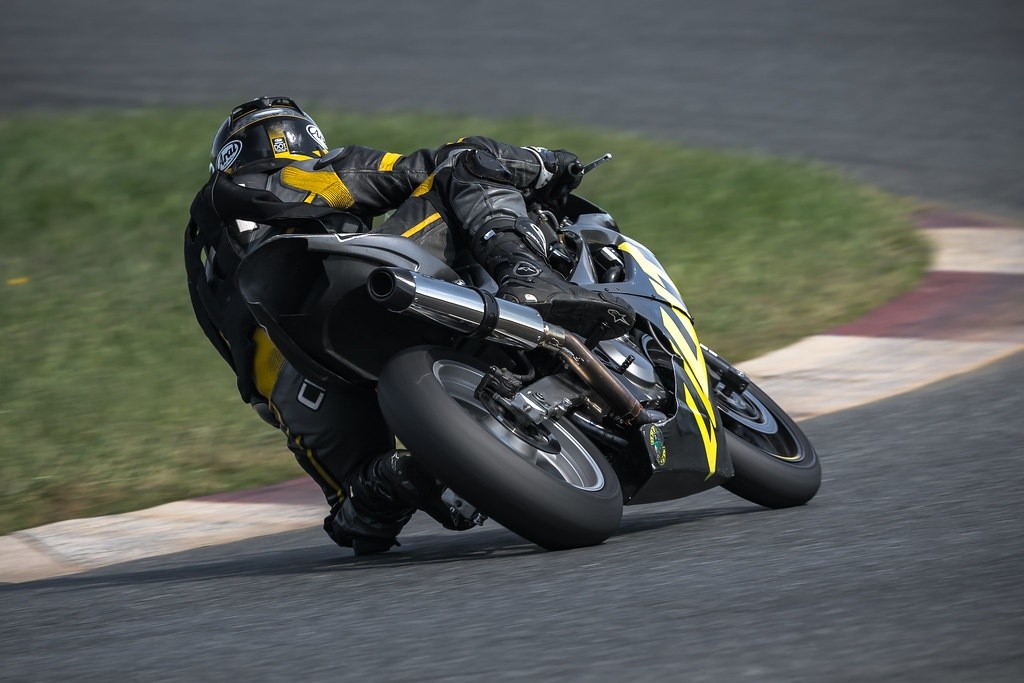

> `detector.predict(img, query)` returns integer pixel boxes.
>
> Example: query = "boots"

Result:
[473,218,636,341]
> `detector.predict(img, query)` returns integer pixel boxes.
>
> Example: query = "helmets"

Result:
[209,97,328,174]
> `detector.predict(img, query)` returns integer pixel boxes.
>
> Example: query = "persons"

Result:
[183,95,635,554]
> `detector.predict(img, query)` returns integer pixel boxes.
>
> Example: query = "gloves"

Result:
[521,147,584,193]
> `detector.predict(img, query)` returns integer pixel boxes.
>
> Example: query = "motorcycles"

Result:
[234,153,823,551]
